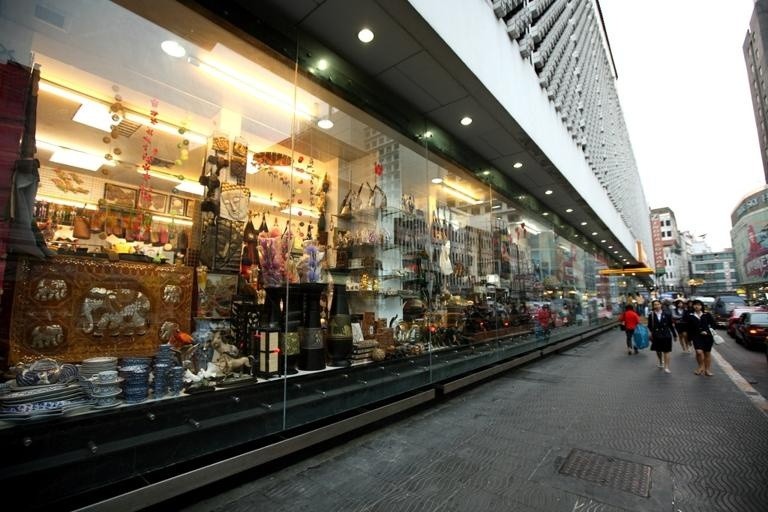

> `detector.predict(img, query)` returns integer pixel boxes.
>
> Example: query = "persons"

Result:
[633,291,645,317]
[606,302,613,322]
[646,300,678,374]
[623,291,627,304]
[740,223,768,265]
[627,292,632,305]
[616,292,623,307]
[465,298,582,358]
[596,302,605,320]
[684,300,718,377]
[617,304,641,355]
[644,299,696,352]
[222,181,252,222]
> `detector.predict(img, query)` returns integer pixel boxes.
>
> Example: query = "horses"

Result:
[217,351,255,383]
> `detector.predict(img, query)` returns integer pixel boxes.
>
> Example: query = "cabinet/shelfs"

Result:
[0,194,623,512]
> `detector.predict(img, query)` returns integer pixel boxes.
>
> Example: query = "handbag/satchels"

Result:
[709,327,724,344]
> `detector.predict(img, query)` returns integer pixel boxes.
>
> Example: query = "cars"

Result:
[463,293,604,331]
[692,295,768,352]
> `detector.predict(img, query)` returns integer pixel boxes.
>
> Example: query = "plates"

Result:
[81,356,118,396]
[87,377,126,409]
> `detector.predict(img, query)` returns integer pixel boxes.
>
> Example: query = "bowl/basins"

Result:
[151,362,183,395]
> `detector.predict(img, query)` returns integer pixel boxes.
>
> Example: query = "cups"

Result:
[92,371,118,406]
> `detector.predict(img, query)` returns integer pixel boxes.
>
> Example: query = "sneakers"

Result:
[696,368,713,376]
[657,361,671,373]
[629,350,639,354]
